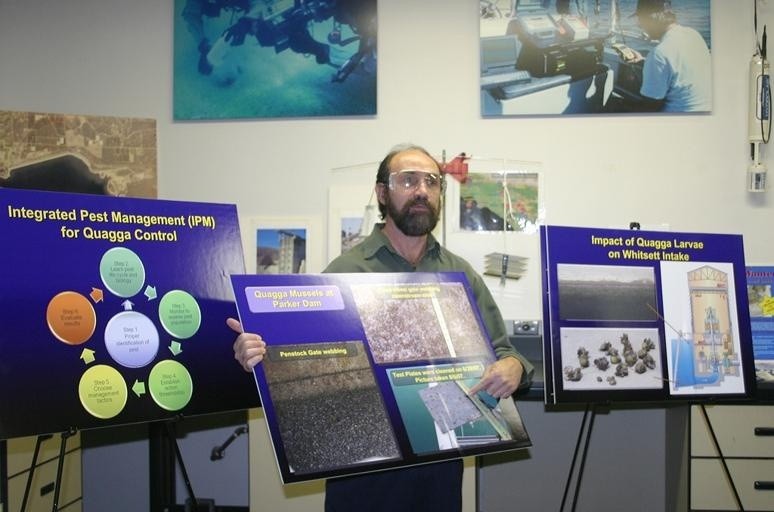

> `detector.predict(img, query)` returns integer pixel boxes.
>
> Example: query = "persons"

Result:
[225,147,535,511]
[619,0,712,113]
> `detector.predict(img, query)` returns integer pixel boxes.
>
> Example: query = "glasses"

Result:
[388,169,447,194]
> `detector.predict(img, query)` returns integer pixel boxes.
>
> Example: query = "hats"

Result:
[627,0,671,19]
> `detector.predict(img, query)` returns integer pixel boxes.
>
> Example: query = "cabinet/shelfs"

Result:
[0,426,83,512]
[682,394,773,510]
